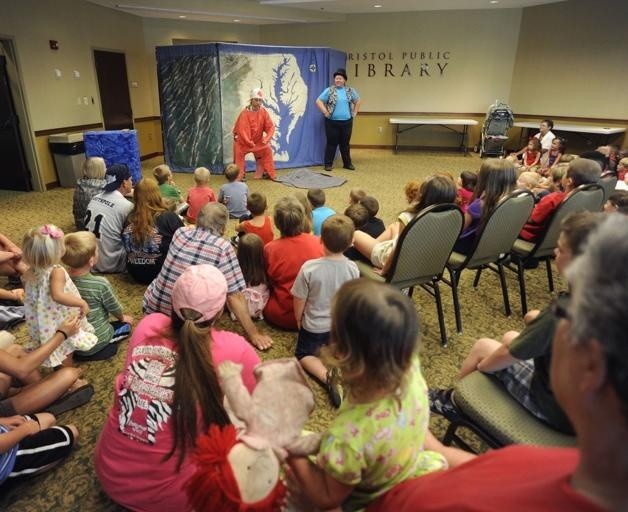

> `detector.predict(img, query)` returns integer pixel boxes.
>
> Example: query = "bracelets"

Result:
[52,327,73,347]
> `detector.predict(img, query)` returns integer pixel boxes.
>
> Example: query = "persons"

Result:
[0,119,626,412]
[1,320,93,418]
[314,68,361,169]
[91,265,265,510]
[1,413,82,493]
[284,280,453,509]
[234,88,275,177]
[363,211,624,511]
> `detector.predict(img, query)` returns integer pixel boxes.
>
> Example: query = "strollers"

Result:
[473,99,513,159]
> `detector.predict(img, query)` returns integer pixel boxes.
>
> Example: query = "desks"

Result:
[513,121,626,160]
[388,117,479,157]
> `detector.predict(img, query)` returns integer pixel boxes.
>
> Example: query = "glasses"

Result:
[551,292,577,326]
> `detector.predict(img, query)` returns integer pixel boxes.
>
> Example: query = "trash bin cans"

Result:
[48,132,86,188]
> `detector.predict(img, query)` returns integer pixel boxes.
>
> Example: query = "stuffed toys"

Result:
[180,355,325,512]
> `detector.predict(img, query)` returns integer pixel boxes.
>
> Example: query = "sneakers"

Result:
[230,236,239,247]
[345,163,354,170]
[325,165,331,170]
[428,389,457,418]
[327,366,343,406]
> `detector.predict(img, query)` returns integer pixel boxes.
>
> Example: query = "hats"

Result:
[333,68,347,80]
[171,264,228,323]
[101,162,130,191]
[248,88,264,100]
